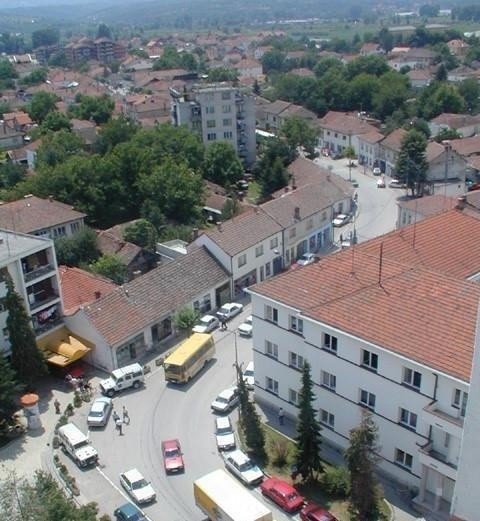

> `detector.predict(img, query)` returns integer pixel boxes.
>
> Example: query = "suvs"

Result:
[121,470,156,505]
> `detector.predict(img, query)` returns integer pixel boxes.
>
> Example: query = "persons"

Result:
[54,398,60,414]
[112,411,120,423]
[116,418,124,436]
[122,406,130,424]
[65,372,84,392]
[221,314,227,329]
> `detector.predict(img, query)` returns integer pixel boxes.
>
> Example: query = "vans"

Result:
[58,423,99,469]
[99,363,145,397]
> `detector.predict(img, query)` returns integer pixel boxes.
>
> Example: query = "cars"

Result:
[333,214,349,226]
[161,439,184,475]
[225,450,264,486]
[216,303,243,321]
[322,147,330,156]
[243,362,255,389]
[87,397,112,427]
[341,236,351,247]
[373,167,406,188]
[192,314,219,333]
[301,502,337,521]
[211,388,239,412]
[238,316,252,337]
[297,252,316,267]
[216,418,237,452]
[260,477,304,513]
[113,504,148,521]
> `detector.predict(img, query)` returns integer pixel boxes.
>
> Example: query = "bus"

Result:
[163,333,215,385]
[193,468,272,521]
